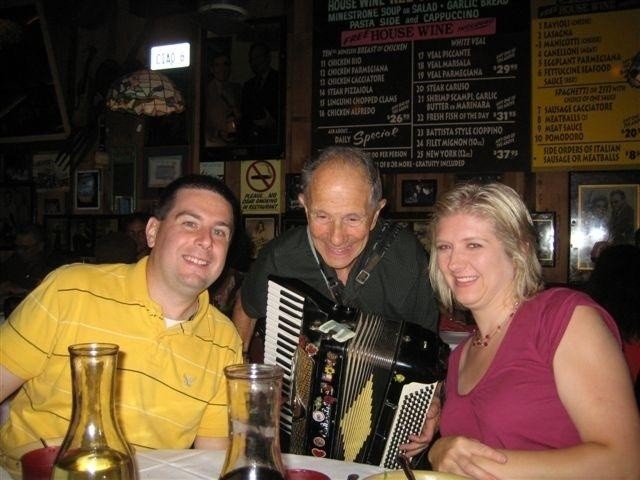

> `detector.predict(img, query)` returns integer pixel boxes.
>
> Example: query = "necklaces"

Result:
[470,301,525,349]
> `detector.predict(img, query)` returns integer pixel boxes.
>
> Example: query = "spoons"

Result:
[396,453,414,479]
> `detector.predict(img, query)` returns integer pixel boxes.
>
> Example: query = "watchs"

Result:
[23,287,28,294]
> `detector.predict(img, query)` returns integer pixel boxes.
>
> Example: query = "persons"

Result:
[0,222,63,295]
[428,181,640,480]
[231,143,441,461]
[586,195,608,232]
[239,46,280,143]
[1,173,244,454]
[608,187,634,241]
[125,212,151,263]
[202,50,246,142]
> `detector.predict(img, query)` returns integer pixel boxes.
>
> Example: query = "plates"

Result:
[285,469,329,480]
[21,446,63,479]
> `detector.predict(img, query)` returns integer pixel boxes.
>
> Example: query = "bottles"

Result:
[49,342,139,480]
[218,363,293,480]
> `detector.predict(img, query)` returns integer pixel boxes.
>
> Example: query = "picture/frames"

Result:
[568,169,640,289]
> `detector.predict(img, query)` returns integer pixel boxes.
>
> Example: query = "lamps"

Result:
[107,67,187,119]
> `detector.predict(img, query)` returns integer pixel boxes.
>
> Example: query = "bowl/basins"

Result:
[365,469,470,480]
